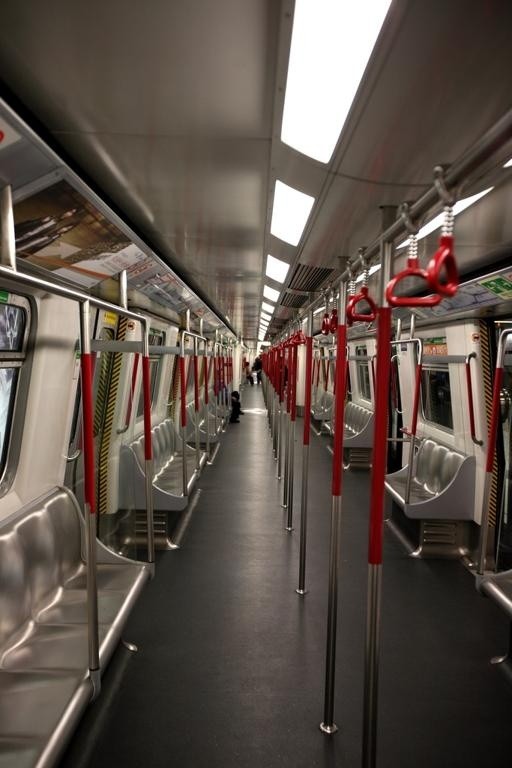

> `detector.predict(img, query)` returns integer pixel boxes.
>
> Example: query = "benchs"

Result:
[310,391,335,420]
[384,437,476,520]
[180,396,224,444]
[118,418,206,511]
[323,401,375,448]
[0,487,148,767]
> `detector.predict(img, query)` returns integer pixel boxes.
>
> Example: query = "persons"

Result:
[251,357,264,385]
[15,203,83,261]
[243,361,254,386]
[231,390,245,424]
